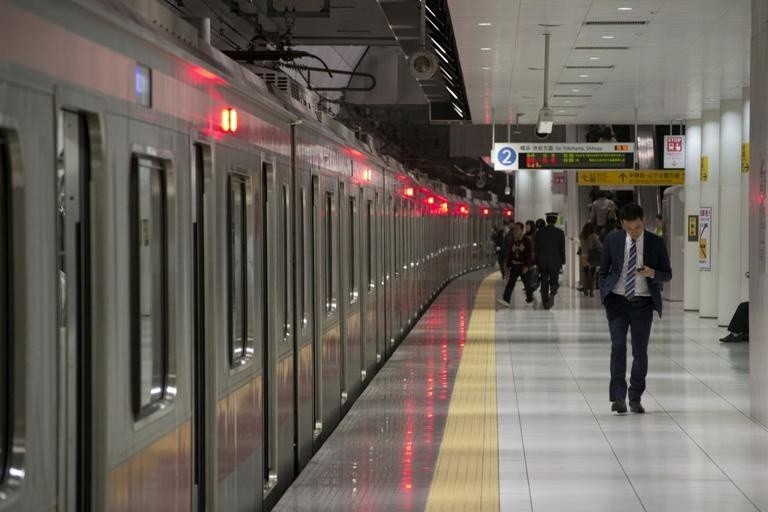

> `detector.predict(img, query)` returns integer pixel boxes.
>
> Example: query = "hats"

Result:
[544,211,558,220]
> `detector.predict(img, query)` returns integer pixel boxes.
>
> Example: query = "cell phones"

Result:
[636,266,644,272]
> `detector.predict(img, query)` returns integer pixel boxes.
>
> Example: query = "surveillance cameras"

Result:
[407,47,439,82]
[504,187,510,196]
[532,109,555,140]
[477,179,485,189]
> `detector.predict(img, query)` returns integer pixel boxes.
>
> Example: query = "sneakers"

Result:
[524,298,533,308]
[496,298,510,309]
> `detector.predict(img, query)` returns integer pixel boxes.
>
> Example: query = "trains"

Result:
[0,0,513,512]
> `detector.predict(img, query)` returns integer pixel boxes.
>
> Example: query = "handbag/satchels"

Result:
[587,246,602,265]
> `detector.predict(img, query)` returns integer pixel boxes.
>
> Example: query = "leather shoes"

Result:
[610,398,628,414]
[628,401,645,415]
[720,333,734,342]
[735,333,749,342]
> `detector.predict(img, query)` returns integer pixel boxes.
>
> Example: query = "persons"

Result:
[599,204,671,413]
[578,187,663,295]
[720,301,749,343]
[492,212,566,310]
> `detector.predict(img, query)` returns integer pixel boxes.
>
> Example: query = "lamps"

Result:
[537,33,554,133]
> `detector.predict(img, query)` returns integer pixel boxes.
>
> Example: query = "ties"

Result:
[625,234,637,305]
